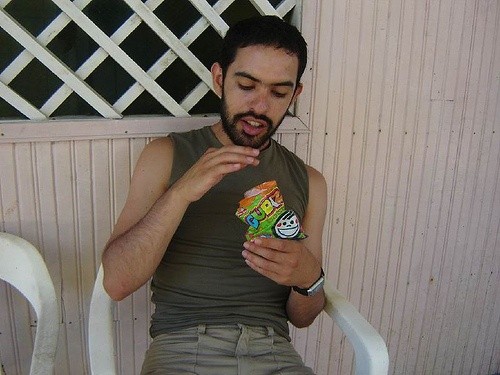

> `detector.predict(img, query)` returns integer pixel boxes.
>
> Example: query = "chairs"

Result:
[88,257,390,375]
[0,233,60,375]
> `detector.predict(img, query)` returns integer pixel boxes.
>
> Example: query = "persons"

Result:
[101,15,329,375]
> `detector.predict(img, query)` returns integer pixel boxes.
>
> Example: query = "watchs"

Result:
[292,268,325,296]
